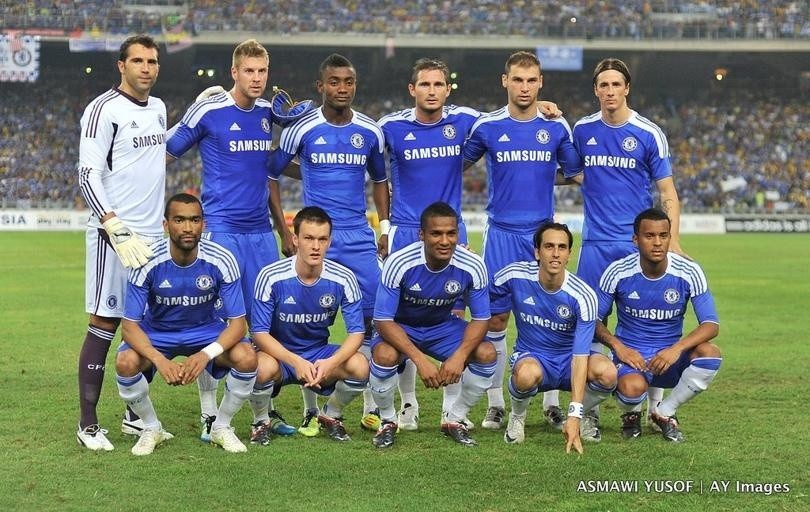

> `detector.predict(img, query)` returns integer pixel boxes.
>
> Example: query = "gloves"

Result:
[103,217,154,270]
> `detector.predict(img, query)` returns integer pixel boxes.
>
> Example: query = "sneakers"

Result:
[544,404,602,443]
[439,411,475,447]
[250,409,350,445]
[76,423,115,452]
[502,413,527,445]
[482,407,507,429]
[121,419,172,456]
[361,404,419,448]
[200,412,247,453]
[621,411,643,440]
[648,408,683,442]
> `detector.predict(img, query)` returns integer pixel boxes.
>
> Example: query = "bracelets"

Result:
[379,221,391,237]
[200,342,223,360]
[568,401,585,420]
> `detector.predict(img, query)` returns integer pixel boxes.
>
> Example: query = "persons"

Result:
[555,59,682,431]
[0,0,808,217]
[370,202,498,447]
[164,39,301,445]
[247,204,370,446]
[595,210,723,442]
[460,51,586,429]
[115,194,258,456]
[269,53,392,436]
[460,220,619,455]
[77,36,224,450]
[363,58,564,433]
